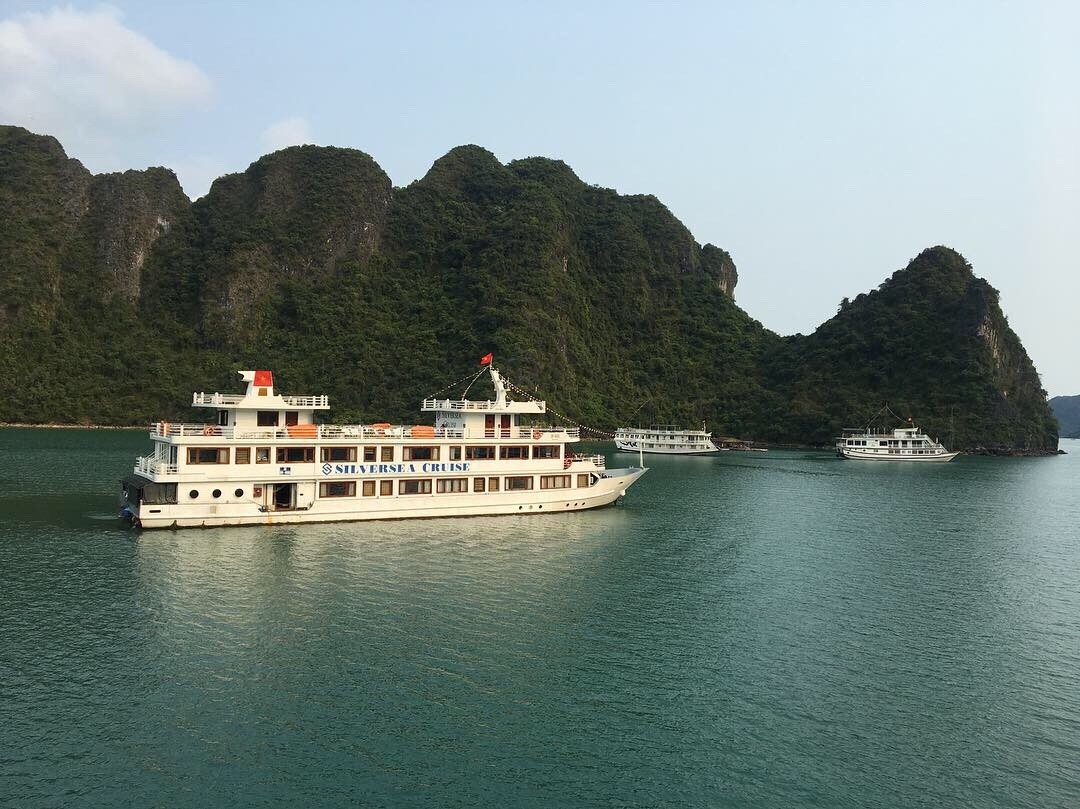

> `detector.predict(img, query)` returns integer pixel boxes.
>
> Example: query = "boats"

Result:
[835,427,960,462]
[120,352,650,531]
[613,427,721,455]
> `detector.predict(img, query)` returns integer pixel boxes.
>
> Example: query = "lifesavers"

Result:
[565,458,572,466]
[204,427,214,436]
[534,431,541,439]
[159,420,170,436]
